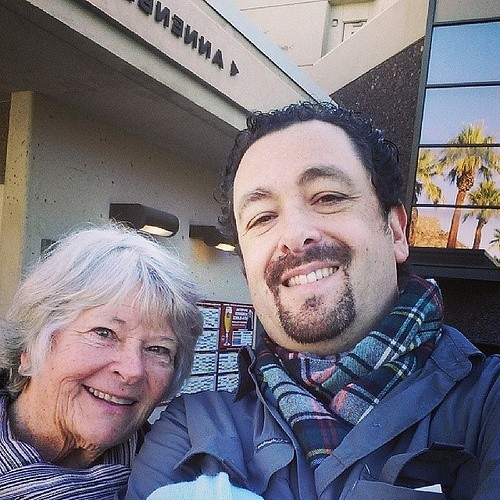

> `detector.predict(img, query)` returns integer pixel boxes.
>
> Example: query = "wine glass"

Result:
[222,307,233,346]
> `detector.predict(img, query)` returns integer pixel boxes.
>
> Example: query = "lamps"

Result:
[189,224,237,255]
[110,202,180,239]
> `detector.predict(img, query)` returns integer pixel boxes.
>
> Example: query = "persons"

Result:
[122,98,499,499]
[1,222,201,500]
